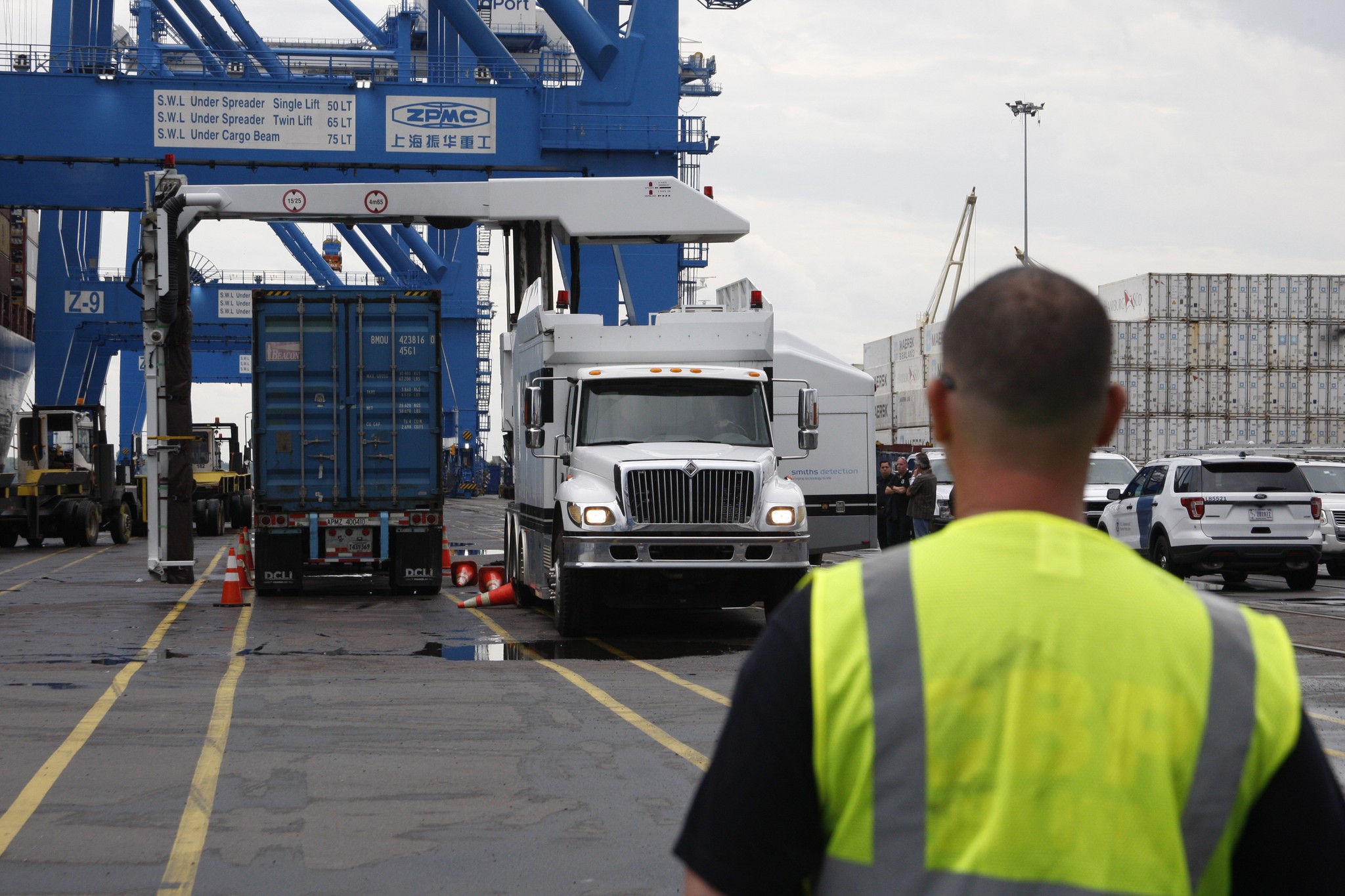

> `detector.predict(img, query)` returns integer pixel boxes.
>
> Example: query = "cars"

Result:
[1082,445,1142,529]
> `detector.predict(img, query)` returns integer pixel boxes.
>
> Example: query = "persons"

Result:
[877,461,899,551]
[907,453,940,541]
[687,390,741,437]
[886,457,914,546]
[677,261,1345,896]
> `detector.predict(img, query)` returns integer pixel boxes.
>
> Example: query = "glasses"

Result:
[897,456,908,465]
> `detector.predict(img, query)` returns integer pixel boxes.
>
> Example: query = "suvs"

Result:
[906,447,956,529]
[1096,452,1325,586]
[1269,453,1345,580]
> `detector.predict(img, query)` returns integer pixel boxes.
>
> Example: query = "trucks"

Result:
[500,278,811,643]
[242,274,446,594]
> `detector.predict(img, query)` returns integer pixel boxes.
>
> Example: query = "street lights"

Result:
[1004,98,1046,269]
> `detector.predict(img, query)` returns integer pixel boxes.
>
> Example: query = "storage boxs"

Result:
[862,272,1345,471]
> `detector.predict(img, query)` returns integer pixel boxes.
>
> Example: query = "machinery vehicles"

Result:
[0,398,142,550]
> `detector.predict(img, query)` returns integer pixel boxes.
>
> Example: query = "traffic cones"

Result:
[243,526,254,571]
[442,525,452,569]
[236,533,254,590]
[213,547,253,608]
[476,566,506,594]
[457,576,528,609]
[450,560,479,588]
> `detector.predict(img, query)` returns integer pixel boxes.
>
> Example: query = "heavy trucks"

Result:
[137,417,252,543]
